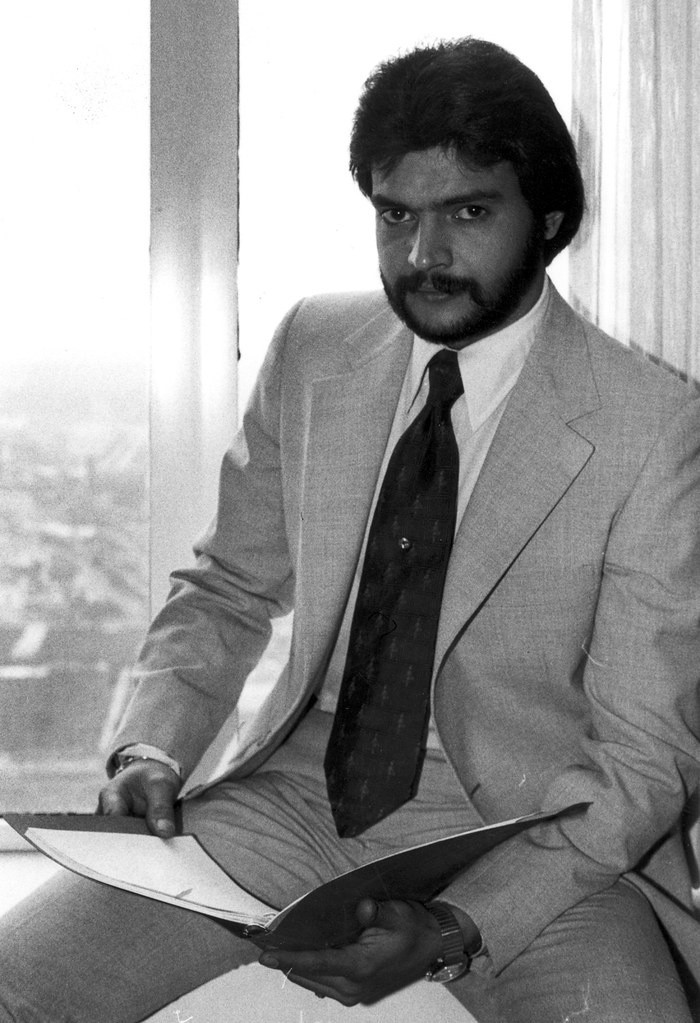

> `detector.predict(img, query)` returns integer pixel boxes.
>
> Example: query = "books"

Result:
[0,801,594,953]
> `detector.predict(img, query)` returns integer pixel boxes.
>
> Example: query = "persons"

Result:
[1,38,700,1023]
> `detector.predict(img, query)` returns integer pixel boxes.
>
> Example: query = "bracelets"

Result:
[114,755,150,775]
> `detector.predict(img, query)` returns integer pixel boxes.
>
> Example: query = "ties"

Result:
[323,350,464,842]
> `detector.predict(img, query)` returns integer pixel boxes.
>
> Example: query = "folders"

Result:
[2,802,589,954]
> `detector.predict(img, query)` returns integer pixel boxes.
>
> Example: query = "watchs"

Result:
[423,902,470,982]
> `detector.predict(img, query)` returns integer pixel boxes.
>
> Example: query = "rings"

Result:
[315,992,325,998]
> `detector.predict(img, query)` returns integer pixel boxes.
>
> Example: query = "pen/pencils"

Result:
[242,925,262,936]
